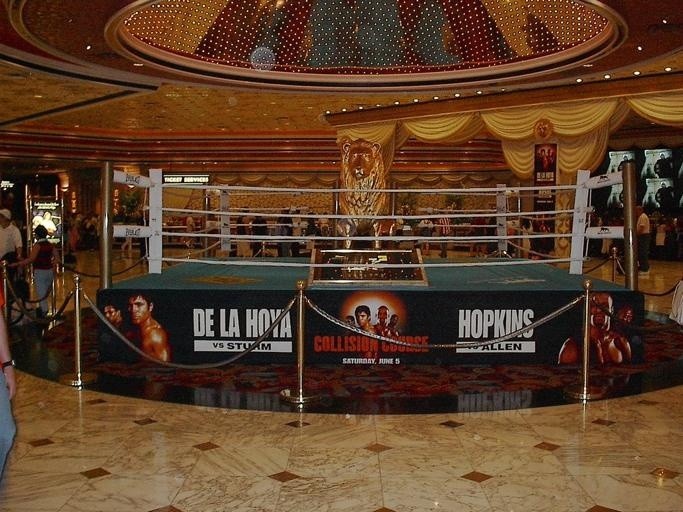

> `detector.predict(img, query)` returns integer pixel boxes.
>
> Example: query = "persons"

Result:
[608,154,676,207]
[1,210,61,317]
[587,206,682,276]
[345,305,401,338]
[559,293,640,365]
[63,207,149,265]
[395,215,534,260]
[0,309,17,473]
[104,292,172,364]
[186,206,322,257]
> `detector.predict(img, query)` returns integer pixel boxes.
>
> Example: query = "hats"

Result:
[0,209,12,220]
[34,225,48,238]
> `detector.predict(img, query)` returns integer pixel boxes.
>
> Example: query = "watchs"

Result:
[2,359,16,368]
